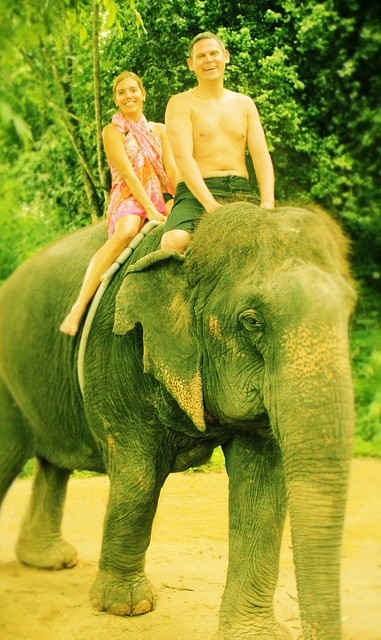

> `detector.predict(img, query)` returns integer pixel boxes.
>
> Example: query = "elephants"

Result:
[0,198,358,635]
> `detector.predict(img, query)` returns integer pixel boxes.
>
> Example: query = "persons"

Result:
[60,69,183,337]
[160,32,275,252]
